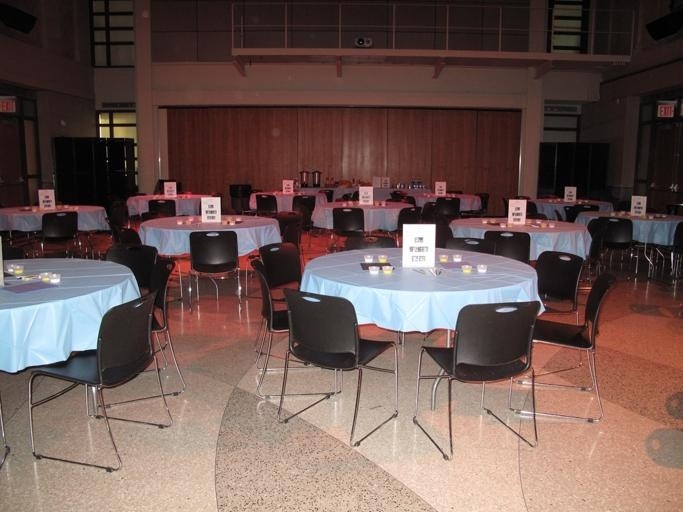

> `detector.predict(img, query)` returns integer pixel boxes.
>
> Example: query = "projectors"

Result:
[354,36,374,47]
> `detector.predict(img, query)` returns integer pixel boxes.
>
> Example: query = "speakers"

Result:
[0,2,37,34]
[646,12,683,41]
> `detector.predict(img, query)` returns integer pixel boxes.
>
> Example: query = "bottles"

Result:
[324,176,342,189]
[350,176,361,187]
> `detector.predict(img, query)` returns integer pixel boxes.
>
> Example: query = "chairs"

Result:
[411,300,542,461]
[94,258,187,410]
[275,288,400,450]
[251,257,343,399]
[508,271,618,424]
[28,287,174,471]
[516,248,586,380]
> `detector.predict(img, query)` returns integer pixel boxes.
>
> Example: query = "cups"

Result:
[395,183,425,190]
[610,210,666,220]
[363,253,489,275]
[482,218,555,228]
[176,215,242,225]
[19,202,79,213]
[5,263,61,287]
